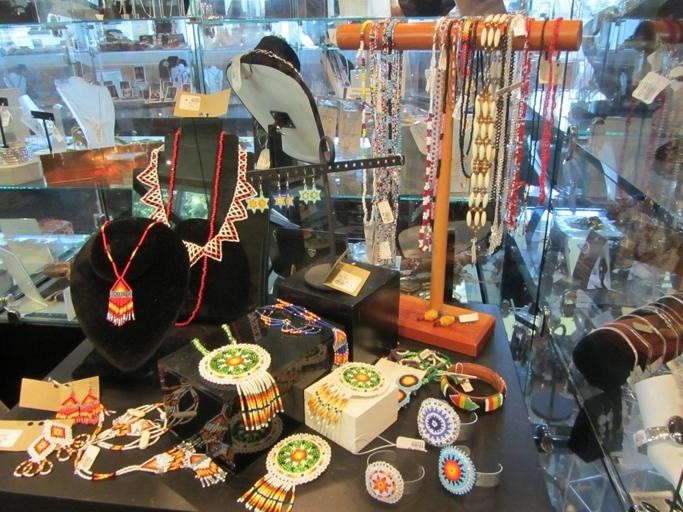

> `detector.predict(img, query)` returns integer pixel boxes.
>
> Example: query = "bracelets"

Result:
[363,451,425,505]
[438,444,503,496]
[415,397,478,447]
[441,363,508,413]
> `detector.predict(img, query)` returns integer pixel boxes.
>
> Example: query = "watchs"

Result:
[633,416,683,455]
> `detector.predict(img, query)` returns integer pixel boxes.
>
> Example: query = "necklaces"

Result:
[417,17,561,254]
[99,220,163,327]
[252,299,349,368]
[14,402,227,486]
[136,145,257,271]
[155,130,229,327]
[191,323,285,432]
[587,295,683,387]
[356,17,404,263]
[6,74,22,90]
[140,0,185,18]
[63,86,108,146]
[253,48,305,79]
